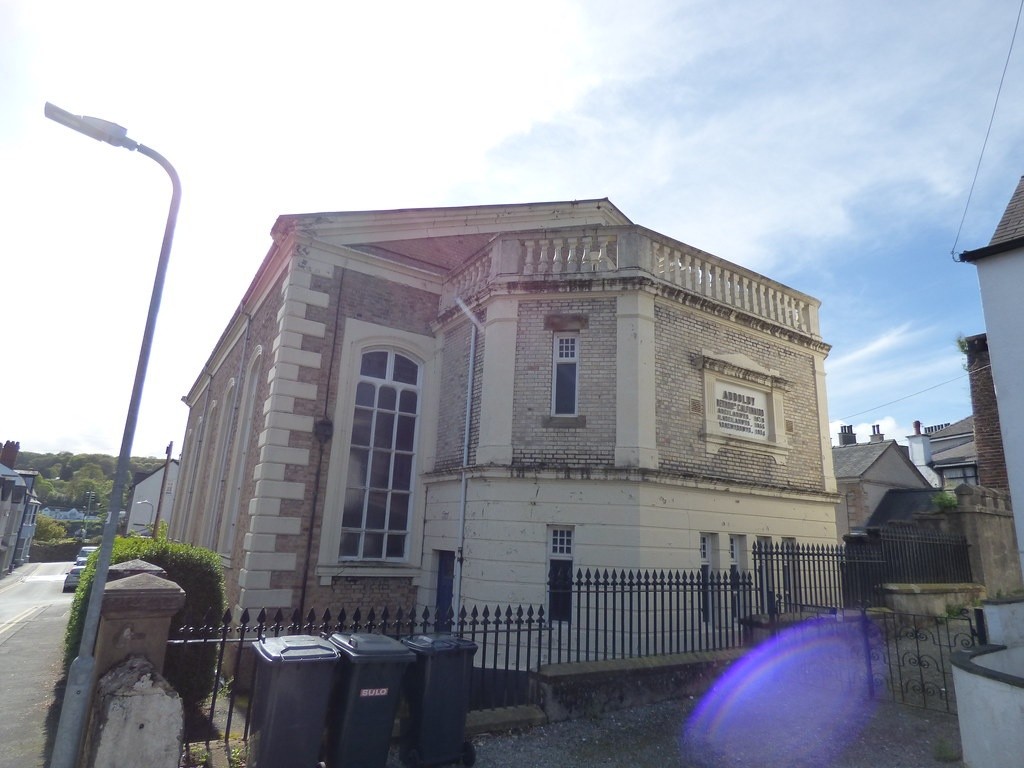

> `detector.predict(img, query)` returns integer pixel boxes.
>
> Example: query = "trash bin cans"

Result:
[247,630,342,768]
[318,631,417,768]
[402,632,476,768]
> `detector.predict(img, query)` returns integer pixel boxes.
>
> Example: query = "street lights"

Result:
[42,98,183,768]
[136,500,156,538]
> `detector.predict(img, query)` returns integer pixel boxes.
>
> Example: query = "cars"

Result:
[63,545,100,593]
